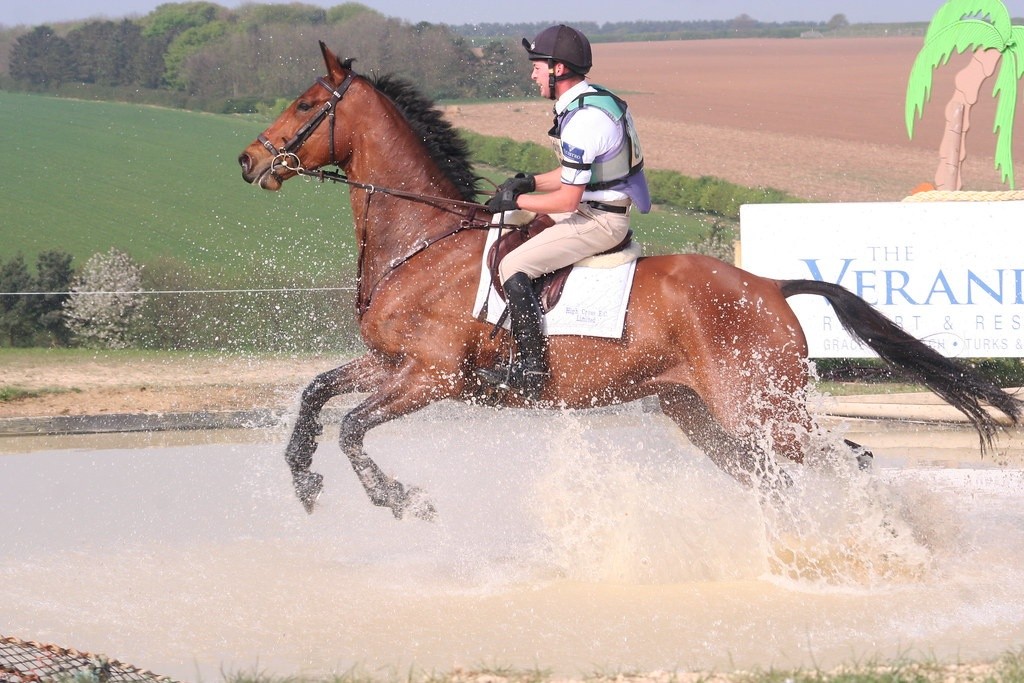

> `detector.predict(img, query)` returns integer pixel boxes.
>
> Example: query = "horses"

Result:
[236,39,1024,523]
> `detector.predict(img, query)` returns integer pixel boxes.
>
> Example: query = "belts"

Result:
[580,200,626,213]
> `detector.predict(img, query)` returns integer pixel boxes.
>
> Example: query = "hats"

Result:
[522,25,593,75]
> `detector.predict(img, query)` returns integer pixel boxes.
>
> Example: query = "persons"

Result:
[472,24,650,402]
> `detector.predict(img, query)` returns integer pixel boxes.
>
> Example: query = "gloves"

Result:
[495,173,535,201]
[483,191,525,215]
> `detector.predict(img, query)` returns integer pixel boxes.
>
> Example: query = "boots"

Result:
[475,272,545,402]
